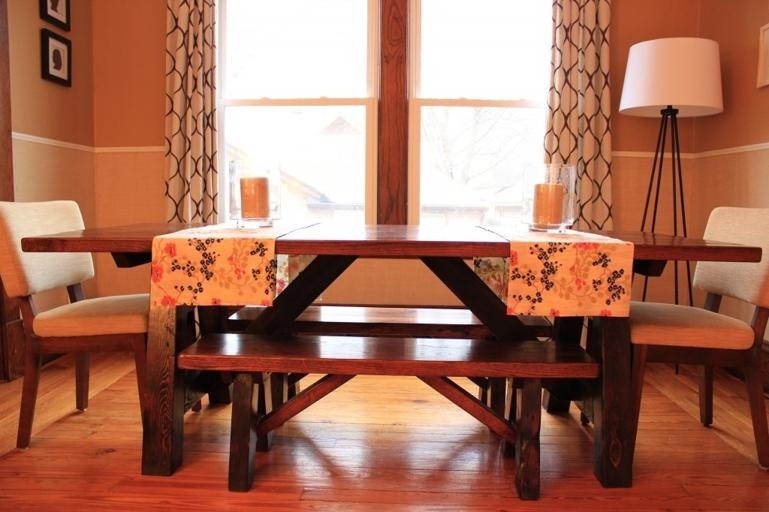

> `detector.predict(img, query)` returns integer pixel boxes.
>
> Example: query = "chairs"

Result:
[1,197,150,449]
[629,207,769,472]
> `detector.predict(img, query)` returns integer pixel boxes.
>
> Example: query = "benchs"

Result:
[178,304,602,500]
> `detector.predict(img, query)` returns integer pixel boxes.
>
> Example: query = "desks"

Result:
[19,225,763,488]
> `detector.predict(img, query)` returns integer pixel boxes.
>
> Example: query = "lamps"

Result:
[618,37,725,308]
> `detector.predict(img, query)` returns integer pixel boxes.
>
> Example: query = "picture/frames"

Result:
[40,0,72,88]
[756,23,769,88]
